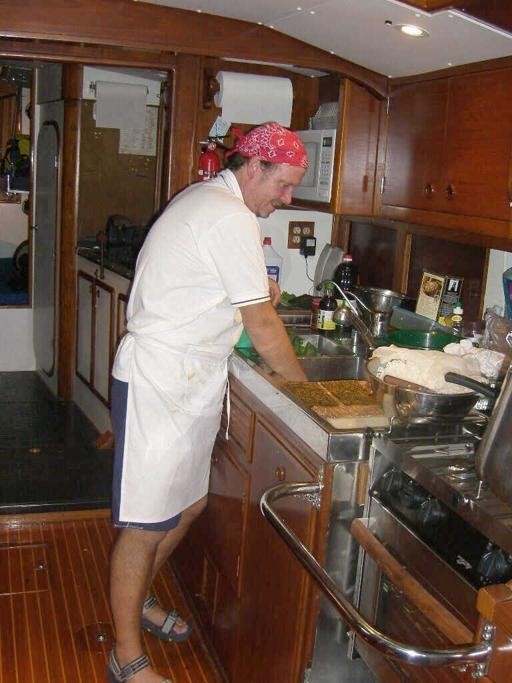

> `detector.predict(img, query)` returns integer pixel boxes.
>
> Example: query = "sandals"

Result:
[107,648,174,683]
[141,596,192,642]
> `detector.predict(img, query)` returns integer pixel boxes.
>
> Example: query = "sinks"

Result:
[239,333,355,356]
[256,356,374,382]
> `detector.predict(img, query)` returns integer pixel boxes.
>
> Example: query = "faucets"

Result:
[314,277,361,319]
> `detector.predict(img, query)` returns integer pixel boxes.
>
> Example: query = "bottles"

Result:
[449,302,465,336]
[316,283,337,338]
[333,251,360,310]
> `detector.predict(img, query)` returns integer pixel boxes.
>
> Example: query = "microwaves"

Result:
[287,129,337,204]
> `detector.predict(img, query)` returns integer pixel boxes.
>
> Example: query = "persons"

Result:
[106,121,308,682]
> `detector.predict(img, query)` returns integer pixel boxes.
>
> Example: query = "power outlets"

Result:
[288,220,317,254]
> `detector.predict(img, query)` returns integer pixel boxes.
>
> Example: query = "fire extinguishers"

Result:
[198,136,230,183]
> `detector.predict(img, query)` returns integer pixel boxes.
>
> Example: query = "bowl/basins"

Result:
[367,356,491,428]
[363,287,413,314]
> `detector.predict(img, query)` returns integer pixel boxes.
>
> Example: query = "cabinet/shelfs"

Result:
[230,420,325,683]
[70,270,113,411]
[380,53,510,227]
[176,390,260,674]
